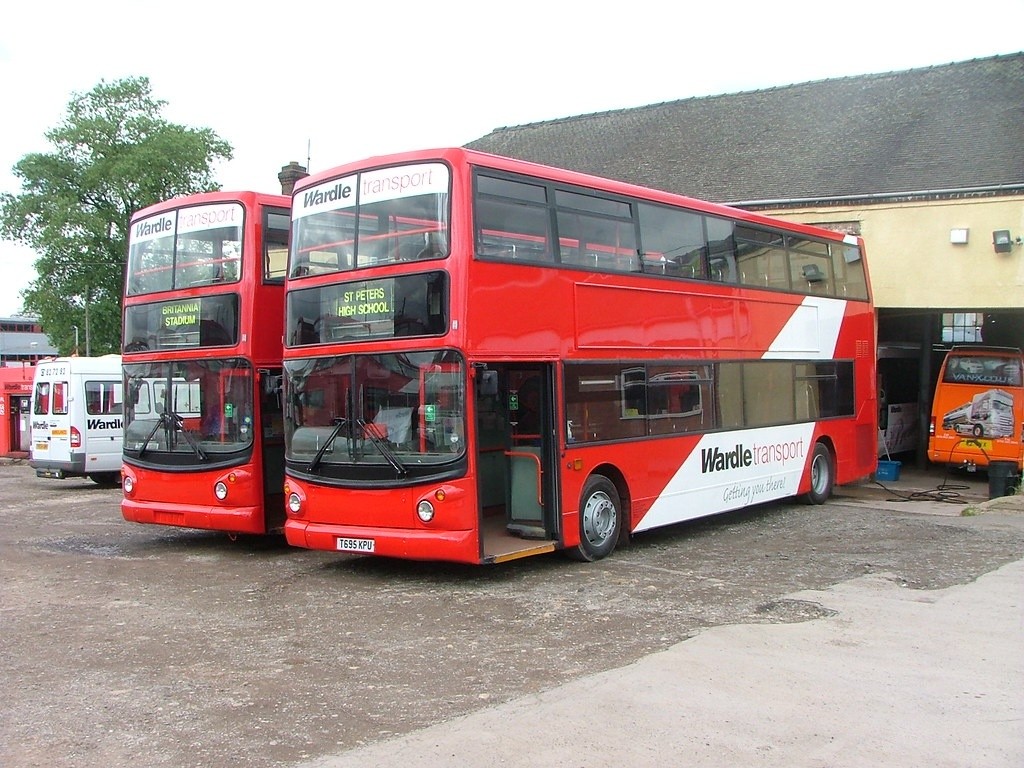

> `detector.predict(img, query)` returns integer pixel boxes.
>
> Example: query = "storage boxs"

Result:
[876,461,901,481]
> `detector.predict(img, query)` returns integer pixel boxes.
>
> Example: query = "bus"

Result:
[265,150,878,568]
[117,191,667,543]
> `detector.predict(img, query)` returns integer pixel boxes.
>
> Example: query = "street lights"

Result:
[70,326,77,355]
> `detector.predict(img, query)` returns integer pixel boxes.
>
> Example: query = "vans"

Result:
[873,340,944,458]
[926,344,1024,473]
[28,353,202,488]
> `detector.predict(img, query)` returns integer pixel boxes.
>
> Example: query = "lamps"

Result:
[993,229,1013,253]
[802,264,825,283]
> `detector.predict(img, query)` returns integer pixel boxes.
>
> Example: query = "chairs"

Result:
[644,262,666,274]
[665,265,695,278]
[584,254,598,266]
[794,281,846,296]
[746,273,768,285]
[712,270,745,284]
[492,244,516,257]
